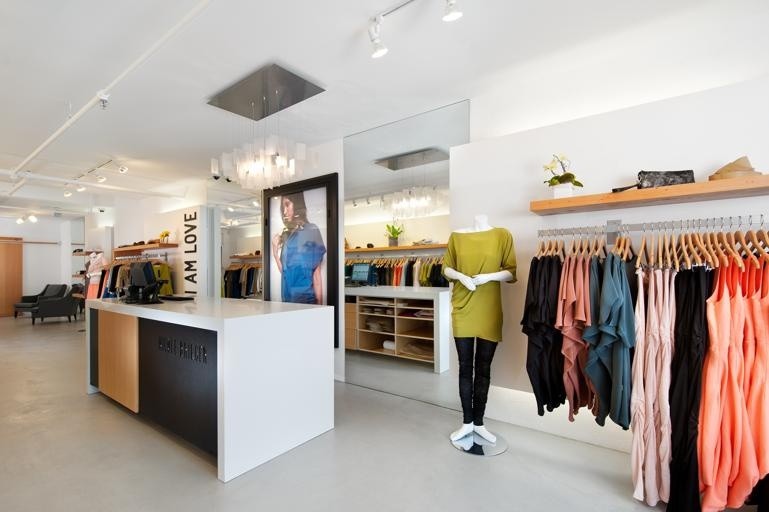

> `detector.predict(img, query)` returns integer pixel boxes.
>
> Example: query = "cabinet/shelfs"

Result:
[70,251,104,299]
[345,301,358,351]
[354,295,434,366]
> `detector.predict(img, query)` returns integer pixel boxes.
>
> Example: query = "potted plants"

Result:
[382,221,404,247]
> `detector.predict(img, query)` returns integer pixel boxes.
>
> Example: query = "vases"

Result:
[553,182,575,197]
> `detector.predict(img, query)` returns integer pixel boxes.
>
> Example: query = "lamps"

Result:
[350,192,386,208]
[367,0,462,62]
[370,148,450,212]
[63,160,129,200]
[207,63,327,192]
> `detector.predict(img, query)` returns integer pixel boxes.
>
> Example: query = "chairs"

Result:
[31,283,83,321]
[72,283,85,314]
[13,280,69,320]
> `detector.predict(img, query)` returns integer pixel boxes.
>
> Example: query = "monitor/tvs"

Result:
[351,263,370,283]
[130,261,156,288]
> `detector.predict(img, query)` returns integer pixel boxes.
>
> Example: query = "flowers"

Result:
[537,149,586,188]
[159,230,171,242]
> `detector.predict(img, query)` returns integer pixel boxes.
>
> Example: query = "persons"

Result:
[270,191,325,304]
[83,250,109,295]
[438,214,518,443]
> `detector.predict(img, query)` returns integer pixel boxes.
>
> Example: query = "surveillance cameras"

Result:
[226,178,233,182]
[213,175,221,180]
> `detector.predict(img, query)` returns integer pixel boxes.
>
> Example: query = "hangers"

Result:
[224,260,262,272]
[346,253,447,268]
[104,255,163,270]
[532,213,767,272]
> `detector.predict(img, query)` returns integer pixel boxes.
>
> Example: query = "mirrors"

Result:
[217,196,266,301]
[341,97,468,416]
